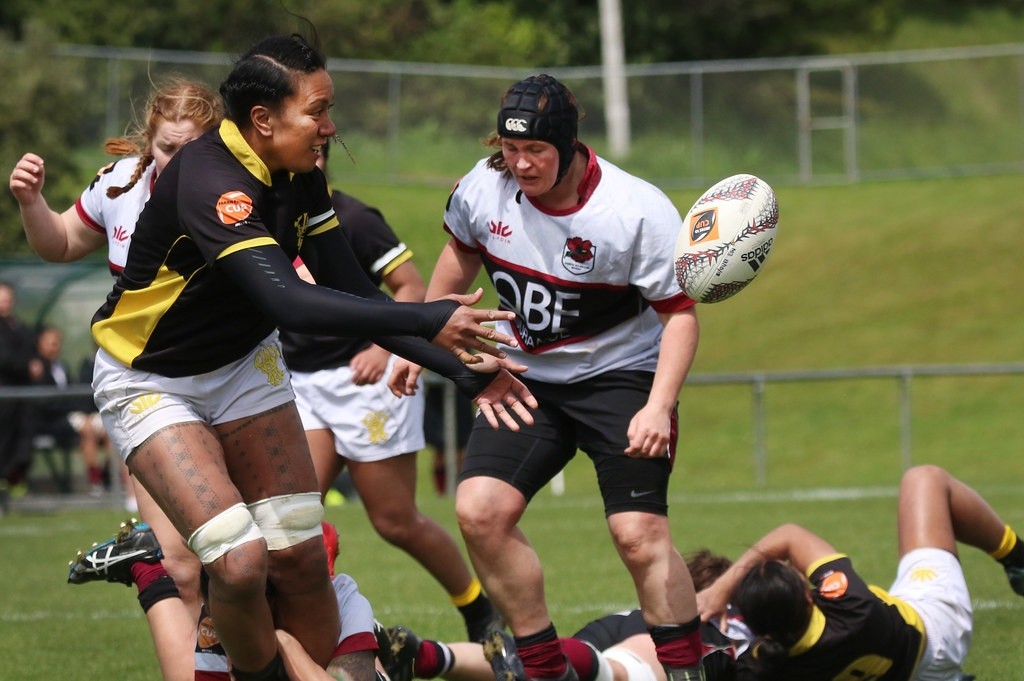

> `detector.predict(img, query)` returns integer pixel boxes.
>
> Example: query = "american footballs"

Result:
[671,174,779,304]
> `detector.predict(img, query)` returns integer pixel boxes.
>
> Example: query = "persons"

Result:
[0,279,139,513]
[9,32,1024,681]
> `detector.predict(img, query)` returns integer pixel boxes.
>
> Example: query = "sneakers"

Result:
[66,519,165,588]
[468,599,506,644]
[376,627,417,681]
[483,630,525,681]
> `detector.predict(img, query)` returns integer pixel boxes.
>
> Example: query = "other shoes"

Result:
[1005,568,1024,597]
